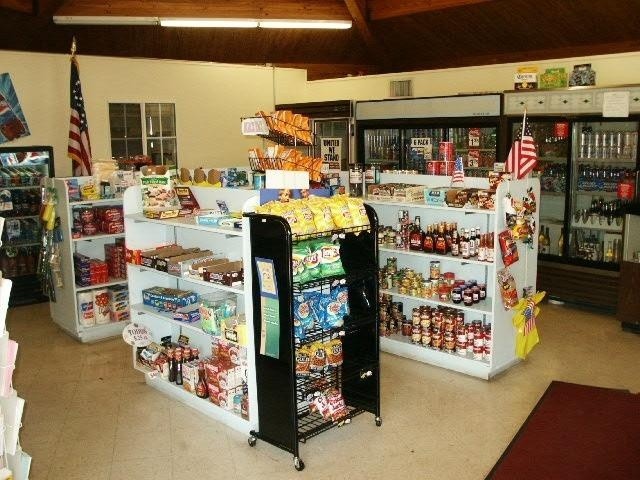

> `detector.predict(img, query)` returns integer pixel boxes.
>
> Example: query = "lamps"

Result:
[53,15,352,30]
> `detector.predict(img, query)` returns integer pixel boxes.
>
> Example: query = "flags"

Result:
[453,156,465,183]
[502,114,539,180]
[65,55,93,177]
[521,298,536,336]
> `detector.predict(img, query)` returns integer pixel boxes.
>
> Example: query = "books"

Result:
[0,216,33,480]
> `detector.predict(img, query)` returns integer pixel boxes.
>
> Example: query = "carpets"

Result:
[483,381,640,480]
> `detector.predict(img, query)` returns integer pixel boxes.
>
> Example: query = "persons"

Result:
[277,189,292,203]
[299,189,311,200]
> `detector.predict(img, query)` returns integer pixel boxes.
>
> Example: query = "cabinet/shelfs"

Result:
[1,215,23,480]
[365,173,539,382]
[124,184,378,463]
[41,174,129,343]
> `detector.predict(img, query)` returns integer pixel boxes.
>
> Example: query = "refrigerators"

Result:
[355,92,502,178]
[0,146,56,308]
[275,100,354,171]
[505,84,640,308]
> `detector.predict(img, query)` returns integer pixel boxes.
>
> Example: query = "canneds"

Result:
[347,163,380,198]
[578,130,640,160]
[377,209,493,363]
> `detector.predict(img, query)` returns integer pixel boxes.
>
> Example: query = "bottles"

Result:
[408,215,494,262]
[367,134,398,161]
[196,362,209,399]
[168,345,199,385]
[558,165,637,262]
[538,225,552,254]
[543,127,567,156]
[0,189,42,277]
